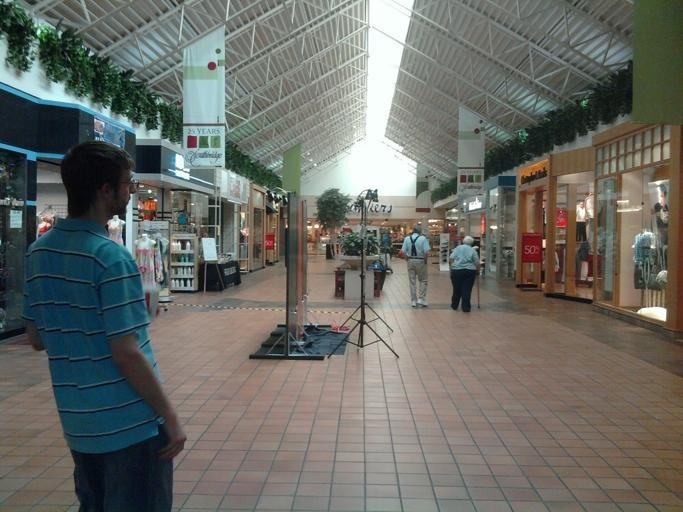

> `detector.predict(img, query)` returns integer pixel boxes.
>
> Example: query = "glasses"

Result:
[120,179,139,190]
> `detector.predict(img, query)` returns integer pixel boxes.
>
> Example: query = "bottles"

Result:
[171,241,193,288]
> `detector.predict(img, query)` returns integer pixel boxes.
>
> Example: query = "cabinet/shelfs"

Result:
[170,236,199,292]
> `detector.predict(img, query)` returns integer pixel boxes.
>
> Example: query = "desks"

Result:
[199,261,241,292]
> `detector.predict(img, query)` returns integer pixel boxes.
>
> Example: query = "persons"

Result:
[138,196,157,221]
[448,235,481,311]
[653,184,668,228]
[403,225,431,308]
[134,231,169,288]
[107,214,126,246]
[36,214,53,238]
[577,202,587,242]
[22,140,186,512]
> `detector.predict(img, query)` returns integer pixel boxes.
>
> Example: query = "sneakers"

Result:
[412,299,428,307]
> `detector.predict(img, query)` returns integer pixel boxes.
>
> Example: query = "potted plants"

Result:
[337,231,382,271]
[311,187,355,259]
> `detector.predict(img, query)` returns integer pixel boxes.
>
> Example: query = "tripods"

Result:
[327,199,400,360]
[339,205,393,333]
